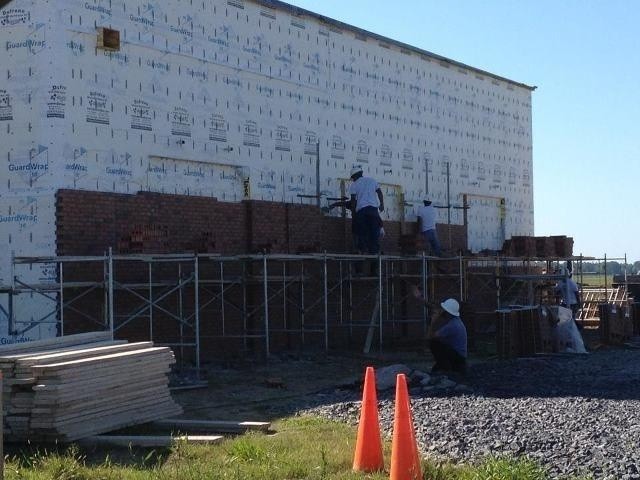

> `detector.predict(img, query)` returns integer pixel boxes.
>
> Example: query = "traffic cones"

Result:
[389,374,423,480]
[353,367,384,472]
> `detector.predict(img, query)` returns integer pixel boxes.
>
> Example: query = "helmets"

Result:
[349,167,364,179]
[422,194,432,202]
[439,297,462,318]
[559,268,571,276]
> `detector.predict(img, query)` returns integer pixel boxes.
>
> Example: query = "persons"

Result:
[555,266,582,321]
[349,166,385,257]
[415,194,442,256]
[327,199,386,253]
[412,285,469,378]
